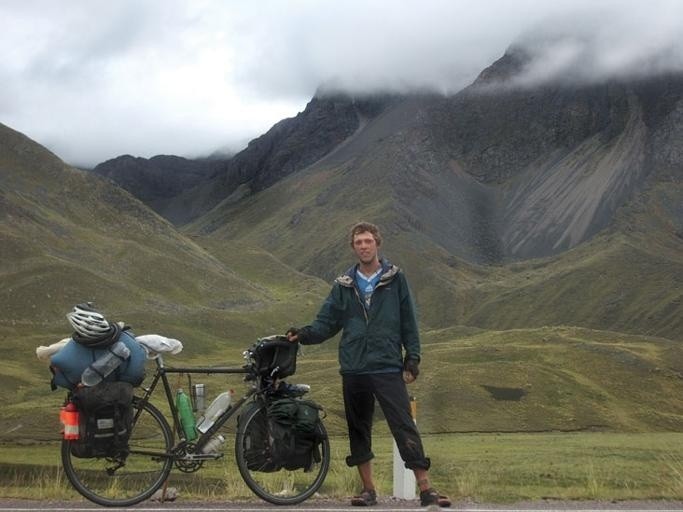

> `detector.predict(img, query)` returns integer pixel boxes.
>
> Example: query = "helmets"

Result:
[67,304,110,337]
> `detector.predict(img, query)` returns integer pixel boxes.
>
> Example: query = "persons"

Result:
[283,223,453,507]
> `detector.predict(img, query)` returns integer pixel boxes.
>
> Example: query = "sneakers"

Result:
[421,487,450,506]
[352,488,376,507]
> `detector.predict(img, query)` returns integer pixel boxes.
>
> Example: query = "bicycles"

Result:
[60,332,331,507]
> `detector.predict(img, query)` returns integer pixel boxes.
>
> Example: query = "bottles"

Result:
[201,436,226,455]
[78,342,130,386]
[193,383,205,410]
[174,388,197,442]
[195,388,236,435]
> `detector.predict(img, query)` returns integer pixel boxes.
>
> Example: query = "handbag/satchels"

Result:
[242,398,320,472]
[71,379,134,458]
